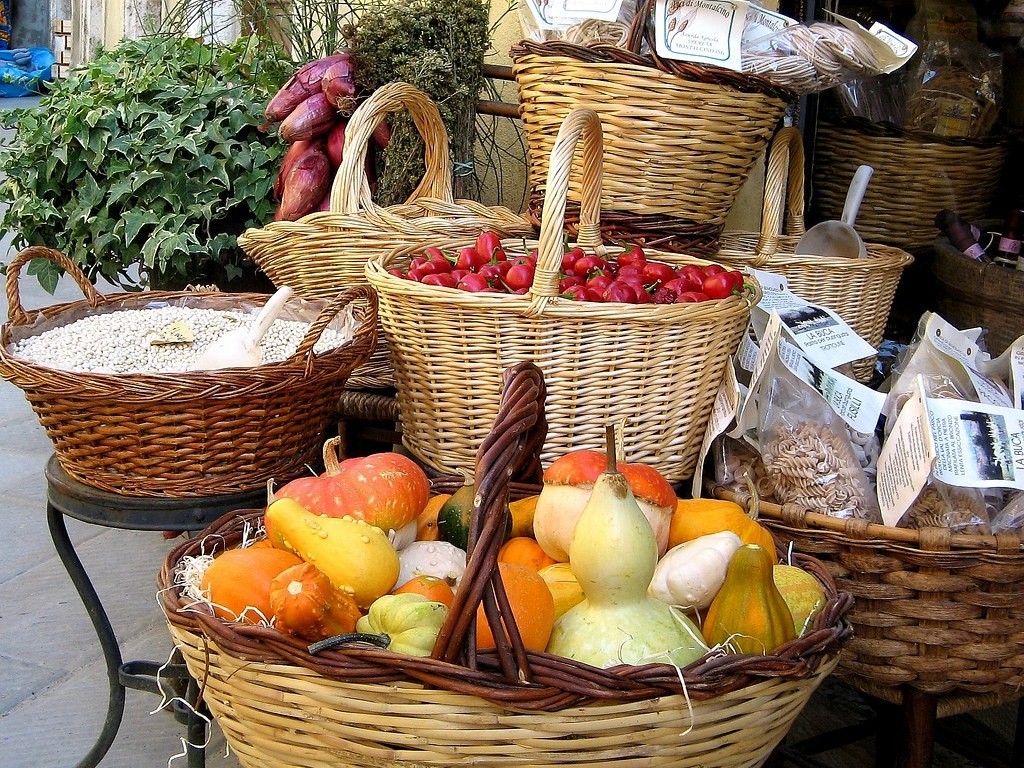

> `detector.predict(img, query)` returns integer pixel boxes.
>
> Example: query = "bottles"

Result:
[994,209,1024,269]
[948,223,991,264]
[1016,245,1024,271]
[935,211,997,258]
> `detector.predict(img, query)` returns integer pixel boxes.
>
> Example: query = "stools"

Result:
[45,451,286,768]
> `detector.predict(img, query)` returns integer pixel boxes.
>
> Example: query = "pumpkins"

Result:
[203,422,825,668]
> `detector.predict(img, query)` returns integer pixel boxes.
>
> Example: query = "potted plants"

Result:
[0,36,299,293]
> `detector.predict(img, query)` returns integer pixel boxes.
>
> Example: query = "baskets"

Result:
[717,128,914,384]
[0,245,379,495]
[701,474,1024,695]
[510,0,794,257]
[366,109,761,495]
[157,361,855,768]
[236,82,532,390]
[936,218,1024,354]
[812,108,1008,251]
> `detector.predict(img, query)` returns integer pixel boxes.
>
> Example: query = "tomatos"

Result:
[389,231,745,304]
